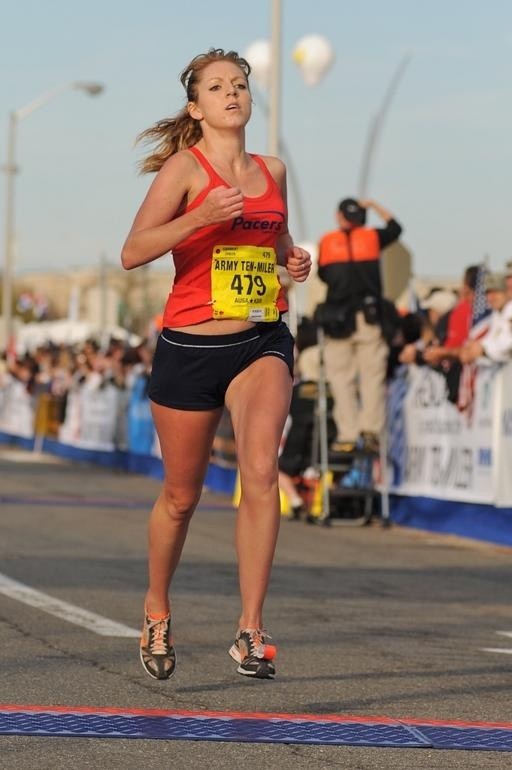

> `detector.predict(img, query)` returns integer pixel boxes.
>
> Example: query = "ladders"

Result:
[306,318,391,529]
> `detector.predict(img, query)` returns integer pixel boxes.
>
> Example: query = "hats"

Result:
[338,197,365,226]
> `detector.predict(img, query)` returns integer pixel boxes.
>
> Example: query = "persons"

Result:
[397,260,512,411]
[279,317,337,517]
[314,196,405,455]
[4,323,157,448]
[114,42,312,686]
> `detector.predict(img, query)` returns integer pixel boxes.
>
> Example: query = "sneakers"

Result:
[226,626,276,680]
[328,429,380,457]
[139,597,177,682]
